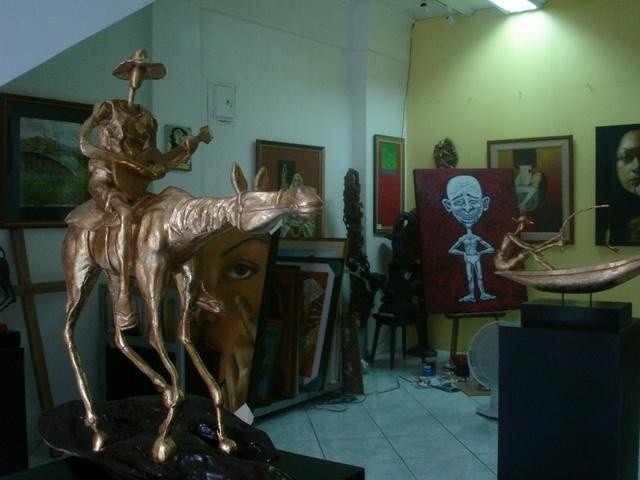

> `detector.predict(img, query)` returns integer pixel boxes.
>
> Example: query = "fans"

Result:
[468,319,520,420]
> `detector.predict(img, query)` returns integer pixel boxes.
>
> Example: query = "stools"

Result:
[370,313,428,370]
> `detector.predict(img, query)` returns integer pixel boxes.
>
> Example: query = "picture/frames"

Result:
[254,139,325,238]
[486,135,575,247]
[373,132,407,236]
[185,220,345,407]
[1,91,96,230]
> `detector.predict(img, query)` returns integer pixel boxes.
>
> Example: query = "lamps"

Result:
[418,0,473,30]
[488,0,545,16]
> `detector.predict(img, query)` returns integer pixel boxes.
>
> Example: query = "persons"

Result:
[78,50,223,330]
[192,227,271,410]
[608,128,640,246]
